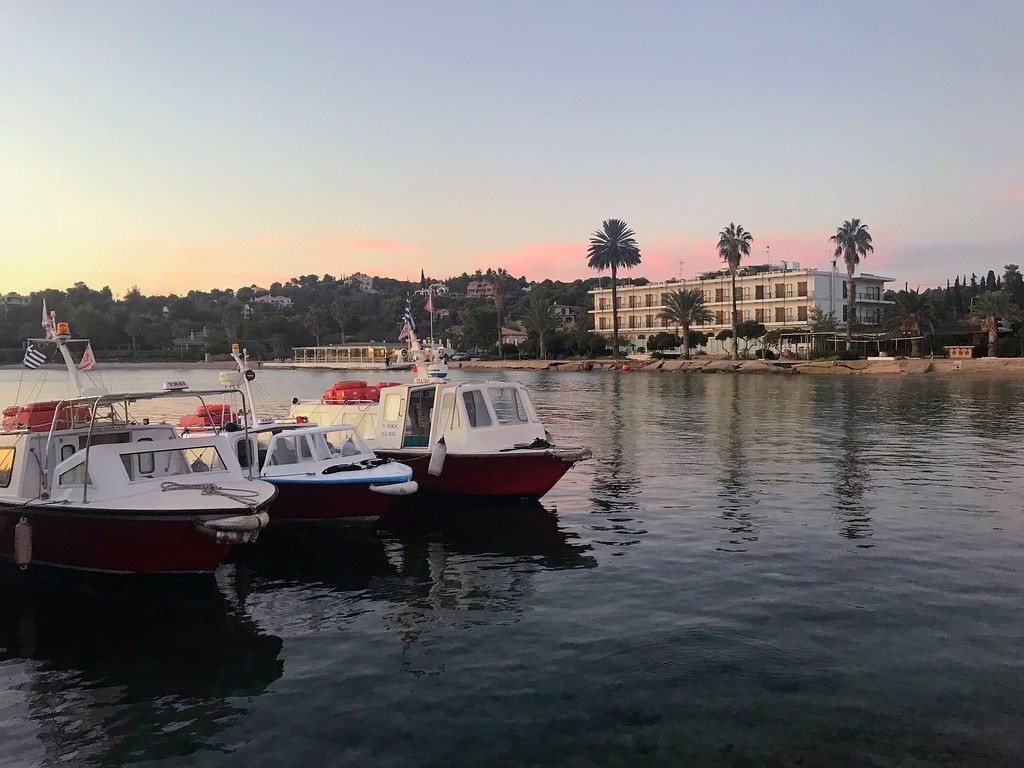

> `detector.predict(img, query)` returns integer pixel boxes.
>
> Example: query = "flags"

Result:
[398,293,416,340]
[78,343,96,370]
[24,341,47,369]
[424,295,436,313]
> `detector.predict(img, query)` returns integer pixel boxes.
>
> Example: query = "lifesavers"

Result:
[196,404,230,417]
[333,380,366,389]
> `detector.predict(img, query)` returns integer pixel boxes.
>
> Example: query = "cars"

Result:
[452,352,471,361]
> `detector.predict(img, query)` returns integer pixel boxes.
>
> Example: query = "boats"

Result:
[171,343,419,532]
[0,298,279,580]
[284,326,592,506]
